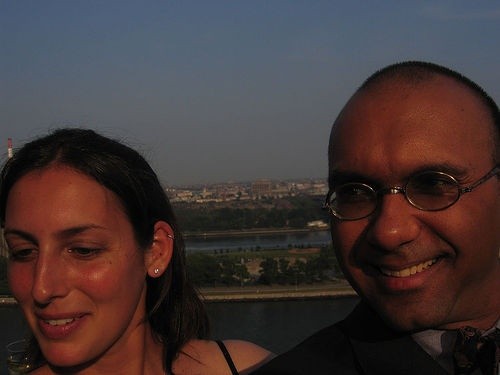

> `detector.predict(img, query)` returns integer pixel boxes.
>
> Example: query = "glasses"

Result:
[323,166,500,221]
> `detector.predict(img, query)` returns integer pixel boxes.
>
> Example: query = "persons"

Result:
[249,60,500,375]
[0,128,278,375]
